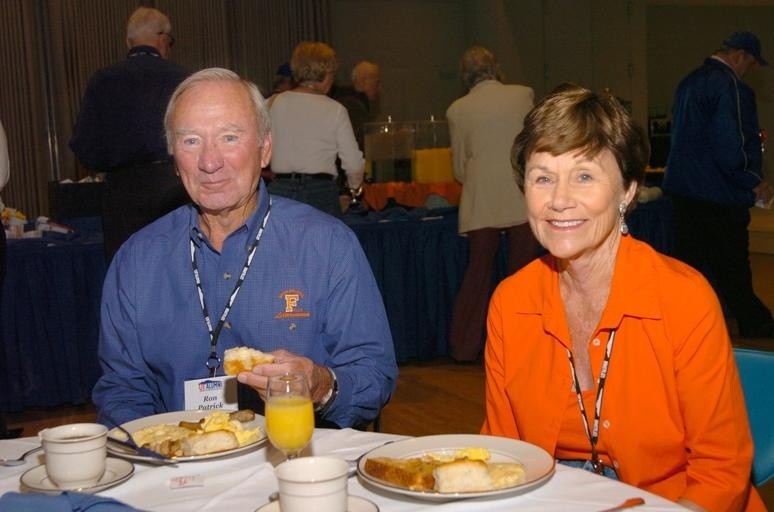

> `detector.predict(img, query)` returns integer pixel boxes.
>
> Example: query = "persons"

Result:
[445,46,541,366]
[332,62,383,185]
[258,41,367,222]
[92,67,398,434]
[481,83,767,511]
[660,30,774,339]
[259,63,297,101]
[69,7,193,247]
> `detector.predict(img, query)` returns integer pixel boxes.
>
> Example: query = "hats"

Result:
[277,60,294,77]
[722,29,770,67]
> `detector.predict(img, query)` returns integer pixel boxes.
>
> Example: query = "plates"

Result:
[358,433,557,500]
[106,408,268,462]
[254,493,380,512]
[20,457,135,492]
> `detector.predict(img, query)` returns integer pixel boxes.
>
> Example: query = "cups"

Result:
[42,424,107,487]
[277,456,349,512]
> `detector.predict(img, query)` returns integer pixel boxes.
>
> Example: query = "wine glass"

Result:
[265,369,315,503]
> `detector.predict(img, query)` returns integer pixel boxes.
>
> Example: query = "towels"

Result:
[0,490,138,511]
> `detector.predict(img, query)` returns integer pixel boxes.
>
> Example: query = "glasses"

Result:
[158,30,175,47]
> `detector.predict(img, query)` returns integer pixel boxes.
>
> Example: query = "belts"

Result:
[272,172,333,180]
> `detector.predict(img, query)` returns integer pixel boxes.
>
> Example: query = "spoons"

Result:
[0,444,42,466]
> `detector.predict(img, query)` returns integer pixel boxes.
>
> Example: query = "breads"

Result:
[365,447,525,495]
[223,346,275,377]
[115,409,264,456]
